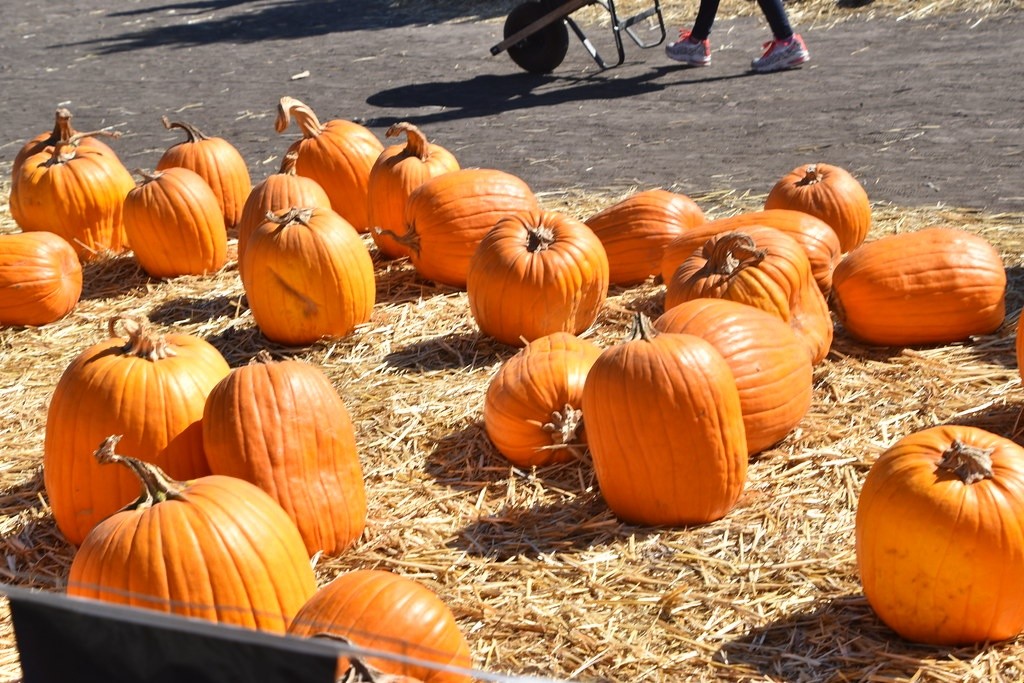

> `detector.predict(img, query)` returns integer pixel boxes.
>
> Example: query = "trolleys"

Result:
[488,0,668,76]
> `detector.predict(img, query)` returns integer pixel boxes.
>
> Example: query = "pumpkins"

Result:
[0,95,1024,683]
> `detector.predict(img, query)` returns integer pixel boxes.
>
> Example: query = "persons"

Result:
[665,0,810,71]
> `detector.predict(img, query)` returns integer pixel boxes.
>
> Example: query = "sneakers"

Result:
[665,28,711,65]
[750,33,810,71]
